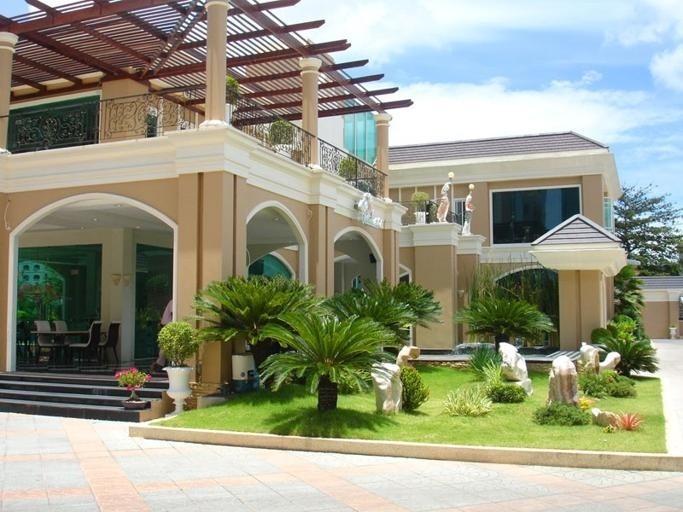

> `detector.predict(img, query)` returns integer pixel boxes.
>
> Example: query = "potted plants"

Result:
[266,114,296,159]
[223,74,234,124]
[155,319,201,419]
[411,191,432,225]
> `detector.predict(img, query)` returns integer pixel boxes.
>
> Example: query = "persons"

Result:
[148,298,172,373]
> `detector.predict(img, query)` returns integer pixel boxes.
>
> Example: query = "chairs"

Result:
[25,318,123,371]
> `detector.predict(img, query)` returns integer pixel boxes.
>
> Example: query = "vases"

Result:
[121,397,151,411]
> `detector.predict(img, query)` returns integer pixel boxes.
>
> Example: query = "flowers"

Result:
[113,366,151,397]
